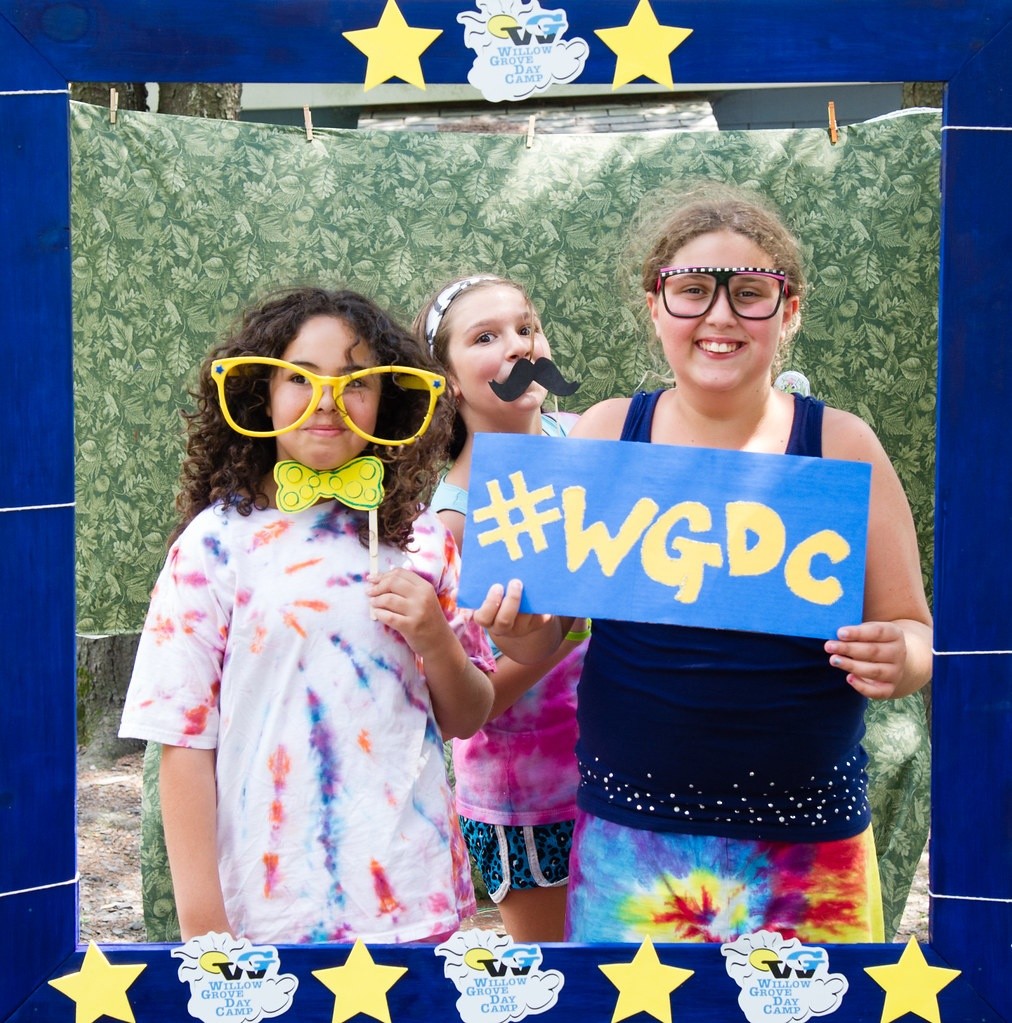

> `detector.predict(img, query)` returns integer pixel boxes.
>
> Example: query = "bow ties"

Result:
[271,453,387,517]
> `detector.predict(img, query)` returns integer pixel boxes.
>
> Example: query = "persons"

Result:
[471,190,933,943]
[406,272,597,942]
[115,281,495,943]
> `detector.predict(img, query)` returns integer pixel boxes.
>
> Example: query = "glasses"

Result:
[646,259,792,321]
[204,347,446,451]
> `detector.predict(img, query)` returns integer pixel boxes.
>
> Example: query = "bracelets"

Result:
[564,627,591,643]
